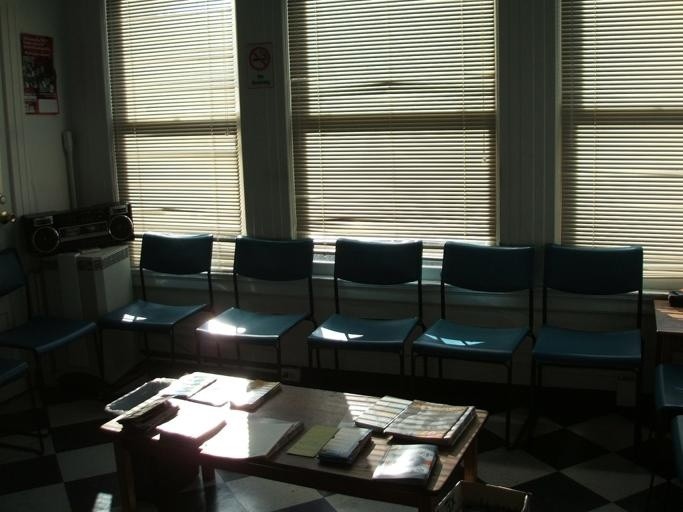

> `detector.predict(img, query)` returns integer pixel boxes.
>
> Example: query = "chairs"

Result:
[100,231,213,386]
[647,361,682,489]
[670,416,682,490]
[400,243,532,434]
[521,242,643,441]
[1,244,100,426]
[198,237,315,386]
[308,241,424,388]
[1,358,46,460]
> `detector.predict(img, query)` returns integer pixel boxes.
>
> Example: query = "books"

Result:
[117,372,371,473]
[372,443,439,486]
[383,397,478,452]
[355,395,405,434]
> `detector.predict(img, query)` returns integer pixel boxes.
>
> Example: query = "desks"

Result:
[652,299,683,363]
[100,369,494,512]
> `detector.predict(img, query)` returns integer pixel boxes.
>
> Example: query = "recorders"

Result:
[21,201,134,258]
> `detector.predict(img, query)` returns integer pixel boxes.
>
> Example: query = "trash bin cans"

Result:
[105,378,178,466]
[433,480,529,512]
